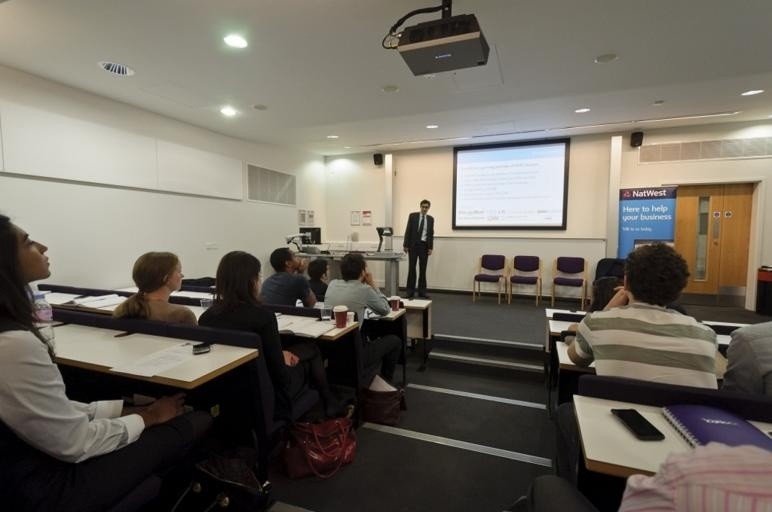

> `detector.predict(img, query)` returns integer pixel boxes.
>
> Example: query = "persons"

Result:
[259,247,317,308]
[196,251,356,421]
[0,214,212,512]
[564,276,626,346]
[305,259,328,302]
[108,251,198,327]
[322,254,402,384]
[551,241,720,478]
[402,199,434,299]
[499,440,771,512]
[719,320,772,401]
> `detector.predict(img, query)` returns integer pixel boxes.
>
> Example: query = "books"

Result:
[662,405,772,451]
[276,316,336,339]
[108,341,201,377]
[70,292,129,309]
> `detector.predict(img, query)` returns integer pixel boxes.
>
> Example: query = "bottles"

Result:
[31,293,55,351]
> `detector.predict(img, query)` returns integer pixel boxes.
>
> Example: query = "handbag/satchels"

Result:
[288,416,359,480]
[357,382,407,426]
[158,456,272,511]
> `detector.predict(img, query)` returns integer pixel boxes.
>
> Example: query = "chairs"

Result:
[473,254,508,305]
[507,253,543,307]
[551,256,588,310]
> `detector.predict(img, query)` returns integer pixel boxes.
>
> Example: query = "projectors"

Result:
[398,13,490,76]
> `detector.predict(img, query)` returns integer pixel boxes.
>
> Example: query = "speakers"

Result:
[631,132,643,147]
[374,154,383,165]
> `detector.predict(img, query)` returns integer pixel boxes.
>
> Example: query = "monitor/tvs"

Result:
[300,228,320,244]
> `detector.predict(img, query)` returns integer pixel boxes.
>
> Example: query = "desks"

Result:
[291,248,404,295]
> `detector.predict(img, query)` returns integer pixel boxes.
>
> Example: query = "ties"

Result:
[417,216,424,244]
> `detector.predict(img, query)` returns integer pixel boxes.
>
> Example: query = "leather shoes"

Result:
[404,295,430,298]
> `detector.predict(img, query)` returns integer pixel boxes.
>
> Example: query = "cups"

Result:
[390,295,401,311]
[199,298,212,311]
[333,304,348,328]
[320,304,332,324]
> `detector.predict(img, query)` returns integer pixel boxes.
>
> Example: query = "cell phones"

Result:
[193,343,210,355]
[611,408,665,441]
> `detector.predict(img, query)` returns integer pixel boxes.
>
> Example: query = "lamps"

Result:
[375,225,394,252]
